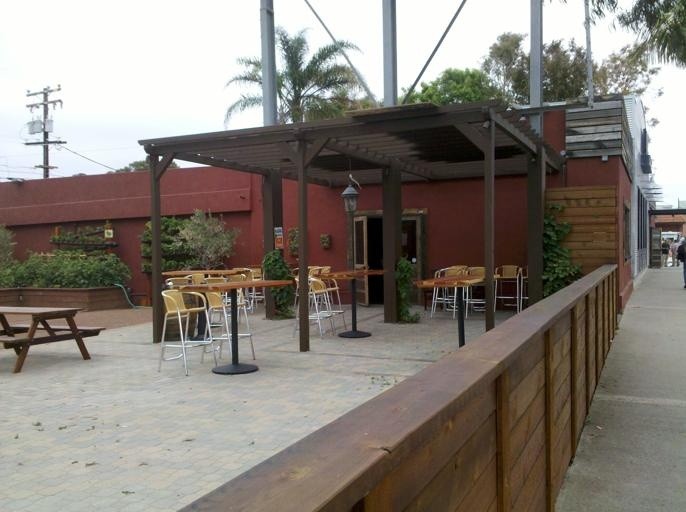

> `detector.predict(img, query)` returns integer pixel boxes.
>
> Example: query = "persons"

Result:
[662,237,686,288]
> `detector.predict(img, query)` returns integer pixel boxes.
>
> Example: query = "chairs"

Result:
[159,264,339,377]
[429,263,523,323]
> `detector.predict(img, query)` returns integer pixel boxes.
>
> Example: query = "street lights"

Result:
[338,174,371,338]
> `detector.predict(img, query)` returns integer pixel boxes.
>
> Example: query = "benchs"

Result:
[0,321,107,354]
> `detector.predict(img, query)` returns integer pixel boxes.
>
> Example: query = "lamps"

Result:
[340,158,360,220]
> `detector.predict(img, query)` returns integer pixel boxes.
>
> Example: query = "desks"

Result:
[313,268,383,339]
[1,305,92,374]
[415,275,485,349]
